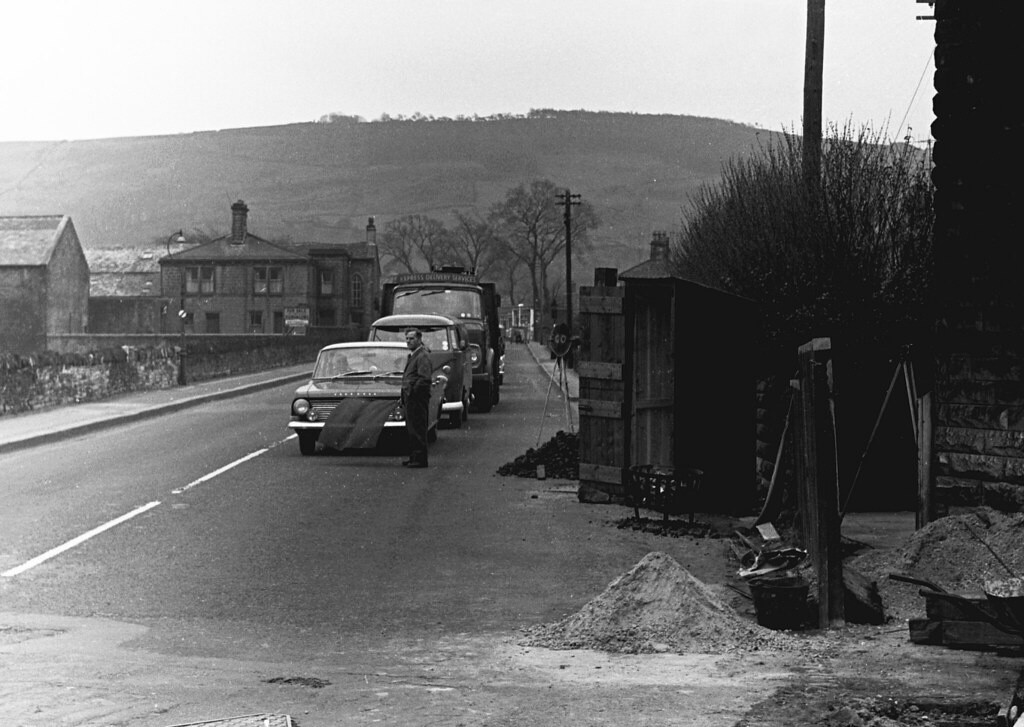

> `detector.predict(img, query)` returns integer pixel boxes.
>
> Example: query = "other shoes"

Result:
[403,459,428,468]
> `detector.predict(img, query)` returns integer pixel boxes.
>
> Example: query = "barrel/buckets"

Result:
[746,575,812,630]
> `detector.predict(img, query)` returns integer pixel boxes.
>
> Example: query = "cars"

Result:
[288,341,451,455]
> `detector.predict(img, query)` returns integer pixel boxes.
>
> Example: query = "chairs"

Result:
[325,354,353,377]
[393,358,408,376]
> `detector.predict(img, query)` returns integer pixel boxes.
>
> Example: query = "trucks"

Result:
[380,263,506,413]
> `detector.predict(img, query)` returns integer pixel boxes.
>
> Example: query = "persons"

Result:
[400,328,431,468]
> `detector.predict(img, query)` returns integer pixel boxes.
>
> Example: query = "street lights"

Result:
[167,228,187,385]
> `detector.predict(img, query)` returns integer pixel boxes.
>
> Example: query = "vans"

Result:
[367,313,472,427]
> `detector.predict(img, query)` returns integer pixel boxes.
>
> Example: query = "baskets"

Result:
[746,576,810,630]
